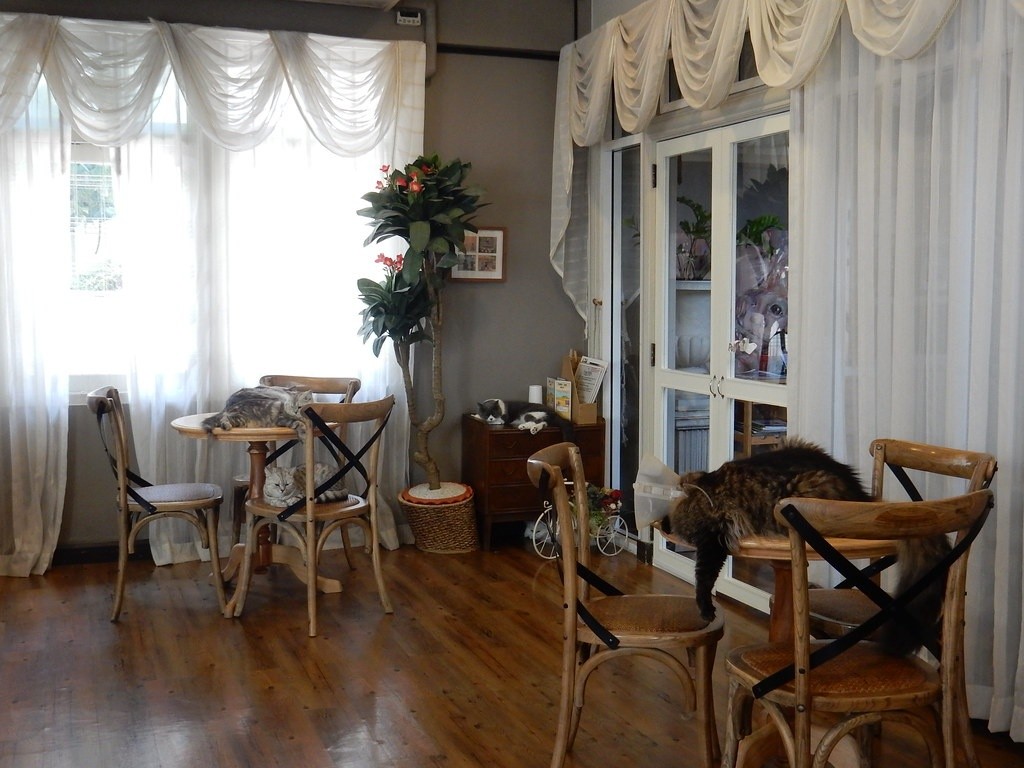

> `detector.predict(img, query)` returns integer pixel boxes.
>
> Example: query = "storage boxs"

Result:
[560,347,598,425]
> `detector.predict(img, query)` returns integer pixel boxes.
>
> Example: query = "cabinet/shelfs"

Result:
[590,130,655,564]
[461,411,605,550]
[649,110,808,615]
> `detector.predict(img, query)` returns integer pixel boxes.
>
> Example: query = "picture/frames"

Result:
[446,223,509,285]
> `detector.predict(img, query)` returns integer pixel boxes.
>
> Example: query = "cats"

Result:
[471,398,573,442]
[201,384,314,444]
[262,462,349,509]
[661,437,951,657]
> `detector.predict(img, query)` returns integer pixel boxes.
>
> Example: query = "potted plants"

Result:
[674,191,715,281]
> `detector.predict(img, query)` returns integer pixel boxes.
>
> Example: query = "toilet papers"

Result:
[528,385,543,405]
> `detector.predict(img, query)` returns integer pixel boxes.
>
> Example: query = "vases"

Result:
[396,480,481,553]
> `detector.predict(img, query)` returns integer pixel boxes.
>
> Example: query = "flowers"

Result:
[354,153,494,491]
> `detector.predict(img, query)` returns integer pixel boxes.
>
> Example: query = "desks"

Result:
[170,410,345,619]
[658,514,942,768]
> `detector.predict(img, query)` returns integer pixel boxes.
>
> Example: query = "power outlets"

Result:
[397,6,421,25]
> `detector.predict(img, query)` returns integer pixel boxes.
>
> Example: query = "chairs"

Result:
[234,394,395,637]
[87,386,227,622]
[527,442,726,768]
[724,489,993,766]
[808,439,999,767]
[735,400,790,457]
[233,375,359,575]
